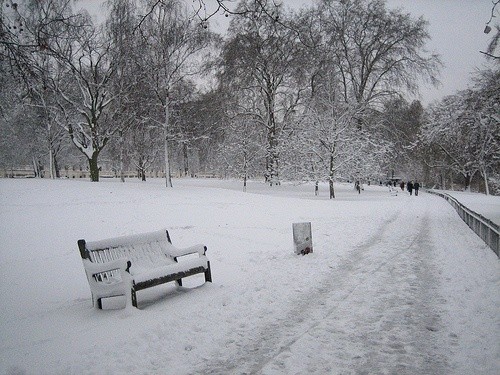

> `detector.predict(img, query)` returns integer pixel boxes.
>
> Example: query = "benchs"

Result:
[6,164,36,178]
[77,227,213,306]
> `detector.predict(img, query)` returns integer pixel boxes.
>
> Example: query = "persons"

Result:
[378,178,420,196]
